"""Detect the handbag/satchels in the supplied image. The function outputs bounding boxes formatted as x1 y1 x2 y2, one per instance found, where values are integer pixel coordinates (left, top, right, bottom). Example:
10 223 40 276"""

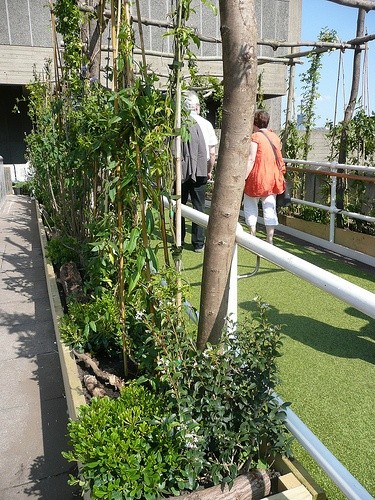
276 185 291 206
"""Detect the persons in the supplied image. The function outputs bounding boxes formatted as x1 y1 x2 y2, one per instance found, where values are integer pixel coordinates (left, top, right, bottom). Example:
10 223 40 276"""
170 89 217 253
244 109 286 246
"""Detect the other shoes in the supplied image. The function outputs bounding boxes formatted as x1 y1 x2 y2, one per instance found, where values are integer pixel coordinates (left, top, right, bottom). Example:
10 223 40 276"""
194 244 204 252
181 241 184 245
